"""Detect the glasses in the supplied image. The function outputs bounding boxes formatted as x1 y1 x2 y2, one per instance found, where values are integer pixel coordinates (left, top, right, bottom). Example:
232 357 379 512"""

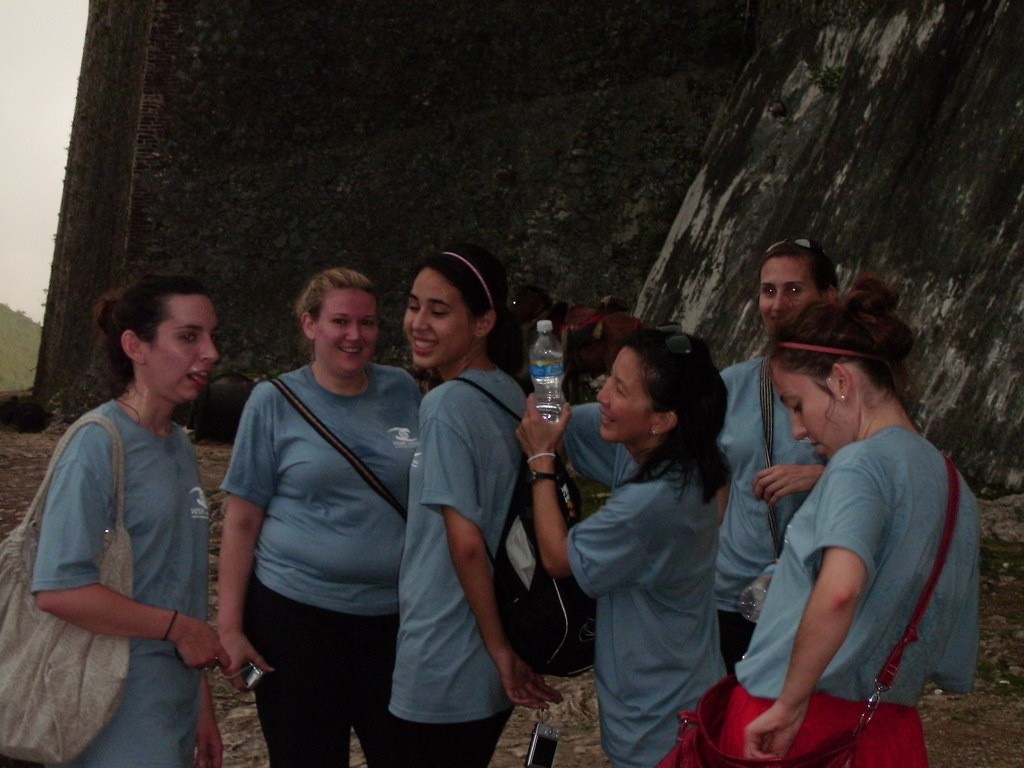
762 237 824 264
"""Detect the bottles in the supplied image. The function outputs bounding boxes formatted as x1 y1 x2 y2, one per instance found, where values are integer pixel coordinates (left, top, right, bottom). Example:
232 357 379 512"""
529 321 566 422
738 559 782 621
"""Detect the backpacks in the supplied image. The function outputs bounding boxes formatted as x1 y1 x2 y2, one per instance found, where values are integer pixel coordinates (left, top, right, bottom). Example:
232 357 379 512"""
450 377 598 677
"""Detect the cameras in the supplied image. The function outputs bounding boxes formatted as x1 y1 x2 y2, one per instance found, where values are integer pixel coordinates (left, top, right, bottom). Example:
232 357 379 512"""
524 723 560 768
242 662 264 690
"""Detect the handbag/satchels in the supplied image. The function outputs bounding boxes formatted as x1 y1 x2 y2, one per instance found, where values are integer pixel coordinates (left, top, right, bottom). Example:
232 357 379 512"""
655 672 856 768
0 415 135 764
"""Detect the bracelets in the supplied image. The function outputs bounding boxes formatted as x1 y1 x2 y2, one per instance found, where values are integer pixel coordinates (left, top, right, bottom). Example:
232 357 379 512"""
526 452 555 464
161 610 179 641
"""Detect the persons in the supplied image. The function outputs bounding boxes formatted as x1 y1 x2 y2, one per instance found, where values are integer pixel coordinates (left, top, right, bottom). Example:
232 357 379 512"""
378 247 564 768
512 319 740 768
685 233 840 673
13 269 235 768
213 267 427 768
723 297 986 768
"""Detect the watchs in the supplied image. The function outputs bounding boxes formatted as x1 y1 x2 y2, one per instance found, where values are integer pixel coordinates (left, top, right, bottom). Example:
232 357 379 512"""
525 469 556 486
